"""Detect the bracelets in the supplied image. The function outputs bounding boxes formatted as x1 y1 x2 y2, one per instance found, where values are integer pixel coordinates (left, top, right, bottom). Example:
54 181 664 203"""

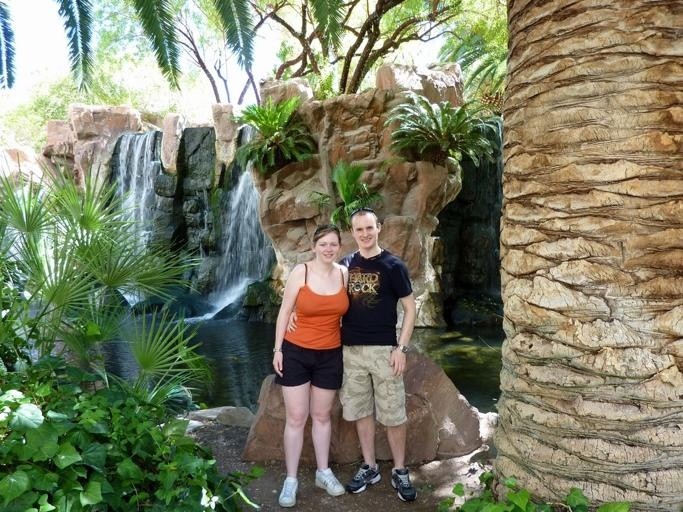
273 348 282 352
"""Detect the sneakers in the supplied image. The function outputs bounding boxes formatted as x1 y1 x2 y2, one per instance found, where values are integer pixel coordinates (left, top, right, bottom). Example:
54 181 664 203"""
391 468 418 502
314 468 346 496
347 464 382 494
278 476 299 508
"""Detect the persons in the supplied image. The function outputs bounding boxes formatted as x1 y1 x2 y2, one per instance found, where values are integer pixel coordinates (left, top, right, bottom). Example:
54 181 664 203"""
285 206 418 503
271 224 350 508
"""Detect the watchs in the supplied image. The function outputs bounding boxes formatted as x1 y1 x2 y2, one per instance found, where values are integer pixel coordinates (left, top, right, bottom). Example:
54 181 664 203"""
396 344 409 353
